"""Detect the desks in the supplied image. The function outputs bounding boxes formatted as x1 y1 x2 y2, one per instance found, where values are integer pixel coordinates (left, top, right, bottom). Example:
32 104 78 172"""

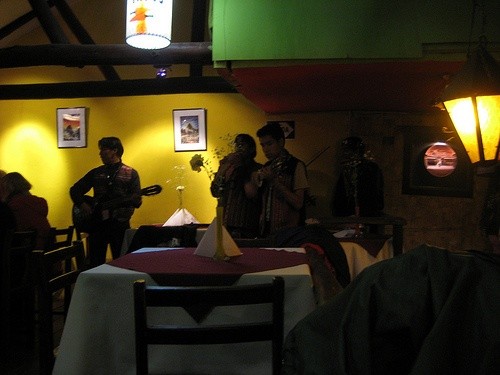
53 235 403 375
314 216 407 258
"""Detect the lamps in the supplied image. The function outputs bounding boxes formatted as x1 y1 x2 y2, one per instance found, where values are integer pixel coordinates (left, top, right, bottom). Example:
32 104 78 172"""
441 10 500 174
150 51 173 79
125 0 173 50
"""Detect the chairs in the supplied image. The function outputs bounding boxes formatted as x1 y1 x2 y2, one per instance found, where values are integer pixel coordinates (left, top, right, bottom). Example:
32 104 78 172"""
0 225 88 375
134 276 285 375
125 225 198 254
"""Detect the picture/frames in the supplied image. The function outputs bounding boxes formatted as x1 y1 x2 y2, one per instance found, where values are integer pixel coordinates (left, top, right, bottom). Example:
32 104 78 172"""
56 106 87 148
401 126 478 199
172 108 207 152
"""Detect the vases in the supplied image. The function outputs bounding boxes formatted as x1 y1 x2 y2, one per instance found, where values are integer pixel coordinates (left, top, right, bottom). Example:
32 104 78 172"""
213 207 230 262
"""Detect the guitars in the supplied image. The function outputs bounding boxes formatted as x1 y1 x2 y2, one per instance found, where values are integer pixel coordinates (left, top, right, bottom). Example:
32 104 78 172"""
72 185 162 232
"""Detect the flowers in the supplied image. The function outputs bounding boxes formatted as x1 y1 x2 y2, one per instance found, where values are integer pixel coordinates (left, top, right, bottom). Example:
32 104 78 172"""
189 133 248 206
165 166 186 209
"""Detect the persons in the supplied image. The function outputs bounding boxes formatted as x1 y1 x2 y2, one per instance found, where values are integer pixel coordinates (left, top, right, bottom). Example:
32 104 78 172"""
70 137 142 271
283 164 500 375
0 173 64 276
251 122 309 236
212 134 266 239
324 134 384 233
